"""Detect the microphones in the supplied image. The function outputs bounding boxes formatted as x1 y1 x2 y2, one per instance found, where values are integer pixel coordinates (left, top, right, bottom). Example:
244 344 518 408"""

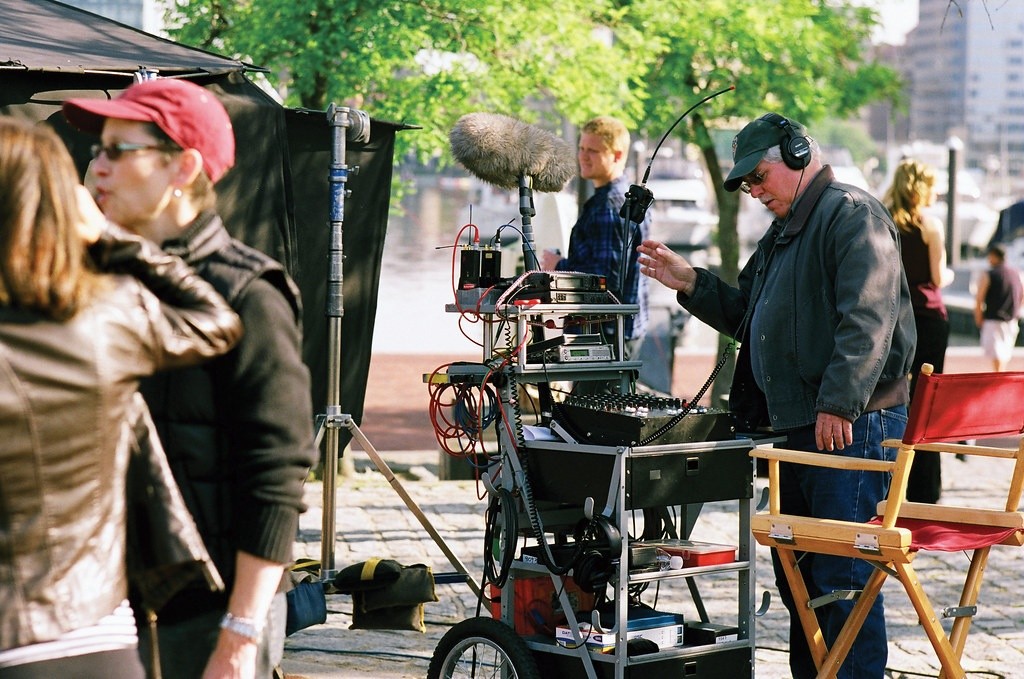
451 114 578 193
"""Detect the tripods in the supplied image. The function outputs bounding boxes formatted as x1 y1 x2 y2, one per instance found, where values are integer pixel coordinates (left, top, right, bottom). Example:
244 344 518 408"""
313 125 492 612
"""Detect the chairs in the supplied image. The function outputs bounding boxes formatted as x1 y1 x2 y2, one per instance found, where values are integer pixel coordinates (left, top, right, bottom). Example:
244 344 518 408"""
749 363 1023 679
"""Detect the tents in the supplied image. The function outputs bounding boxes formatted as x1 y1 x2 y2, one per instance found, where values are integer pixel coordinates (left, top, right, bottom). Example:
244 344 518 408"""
0 0 423 471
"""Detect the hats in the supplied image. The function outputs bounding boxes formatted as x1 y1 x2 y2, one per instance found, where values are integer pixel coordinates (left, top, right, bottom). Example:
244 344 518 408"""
63 79 238 186
723 114 802 192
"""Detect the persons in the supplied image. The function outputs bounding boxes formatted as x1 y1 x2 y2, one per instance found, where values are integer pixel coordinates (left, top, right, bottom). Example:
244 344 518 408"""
973 245 1024 377
635 112 915 679
539 116 652 403
877 160 954 501
0 74 318 679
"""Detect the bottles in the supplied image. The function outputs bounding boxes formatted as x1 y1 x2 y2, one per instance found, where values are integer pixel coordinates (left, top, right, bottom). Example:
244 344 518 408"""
632 547 683 573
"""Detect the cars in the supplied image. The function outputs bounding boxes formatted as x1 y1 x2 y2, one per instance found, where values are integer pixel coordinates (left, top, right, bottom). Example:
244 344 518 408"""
938 255 1023 347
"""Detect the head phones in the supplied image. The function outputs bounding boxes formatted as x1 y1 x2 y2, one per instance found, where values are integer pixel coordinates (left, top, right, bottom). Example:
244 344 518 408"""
760 112 812 170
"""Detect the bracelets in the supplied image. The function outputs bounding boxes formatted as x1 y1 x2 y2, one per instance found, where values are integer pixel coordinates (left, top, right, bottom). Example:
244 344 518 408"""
219 611 265 642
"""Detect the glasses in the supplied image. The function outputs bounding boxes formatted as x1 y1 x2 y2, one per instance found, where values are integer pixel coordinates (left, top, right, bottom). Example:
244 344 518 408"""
90 143 185 160
740 154 780 194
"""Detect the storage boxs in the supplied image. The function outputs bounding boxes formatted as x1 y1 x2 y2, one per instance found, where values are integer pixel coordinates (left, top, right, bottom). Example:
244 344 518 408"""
631 538 738 567
556 627 616 654
